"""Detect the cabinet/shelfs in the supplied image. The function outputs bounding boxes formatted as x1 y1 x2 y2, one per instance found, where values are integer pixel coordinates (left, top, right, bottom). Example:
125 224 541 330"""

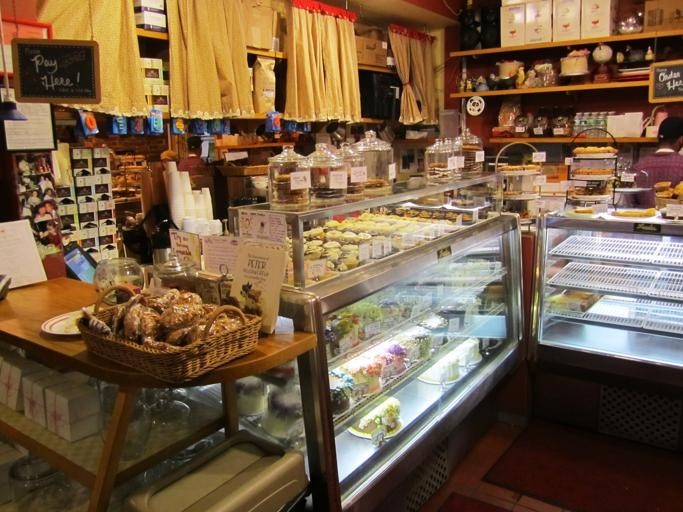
0 1 682 512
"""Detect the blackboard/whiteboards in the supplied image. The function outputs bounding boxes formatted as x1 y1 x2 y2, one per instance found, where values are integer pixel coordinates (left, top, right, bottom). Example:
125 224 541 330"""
11 38 101 104
648 59 683 104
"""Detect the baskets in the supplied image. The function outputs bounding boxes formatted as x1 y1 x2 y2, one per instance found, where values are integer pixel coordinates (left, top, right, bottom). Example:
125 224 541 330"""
75 282 264 387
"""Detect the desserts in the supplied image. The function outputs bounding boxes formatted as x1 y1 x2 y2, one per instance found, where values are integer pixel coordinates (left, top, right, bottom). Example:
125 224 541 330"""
288 204 491 285
288 259 491 447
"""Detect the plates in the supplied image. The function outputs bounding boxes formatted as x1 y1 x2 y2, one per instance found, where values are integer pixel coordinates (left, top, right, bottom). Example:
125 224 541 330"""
42 311 82 336
609 188 655 209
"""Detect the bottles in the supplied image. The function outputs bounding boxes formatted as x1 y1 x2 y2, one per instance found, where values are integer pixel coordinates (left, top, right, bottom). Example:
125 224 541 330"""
268 127 484 209
531 104 616 139
94 254 196 304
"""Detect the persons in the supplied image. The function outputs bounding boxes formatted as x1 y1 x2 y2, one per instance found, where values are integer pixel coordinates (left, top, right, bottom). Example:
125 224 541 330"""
121 202 170 266
248 124 275 164
176 136 226 194
18 157 62 252
623 115 682 209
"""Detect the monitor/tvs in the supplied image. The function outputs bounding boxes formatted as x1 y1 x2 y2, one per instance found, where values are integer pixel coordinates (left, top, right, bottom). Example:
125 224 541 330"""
63 243 97 284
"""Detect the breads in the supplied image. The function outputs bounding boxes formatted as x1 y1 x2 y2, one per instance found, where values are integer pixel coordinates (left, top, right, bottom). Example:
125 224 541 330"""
112 287 252 345
